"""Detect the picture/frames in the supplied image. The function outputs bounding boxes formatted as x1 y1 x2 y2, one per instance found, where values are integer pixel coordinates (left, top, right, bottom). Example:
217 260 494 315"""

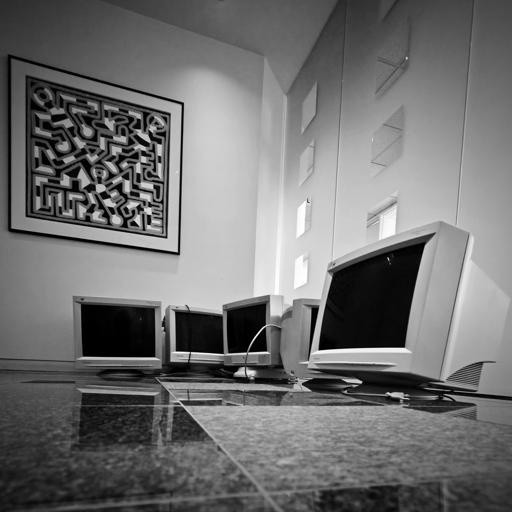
5 53 186 255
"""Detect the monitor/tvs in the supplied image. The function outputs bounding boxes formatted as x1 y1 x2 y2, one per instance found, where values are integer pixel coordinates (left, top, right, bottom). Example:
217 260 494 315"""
71 295 163 380
162 304 228 377
307 220 512 400
278 297 353 391
222 294 300 385
